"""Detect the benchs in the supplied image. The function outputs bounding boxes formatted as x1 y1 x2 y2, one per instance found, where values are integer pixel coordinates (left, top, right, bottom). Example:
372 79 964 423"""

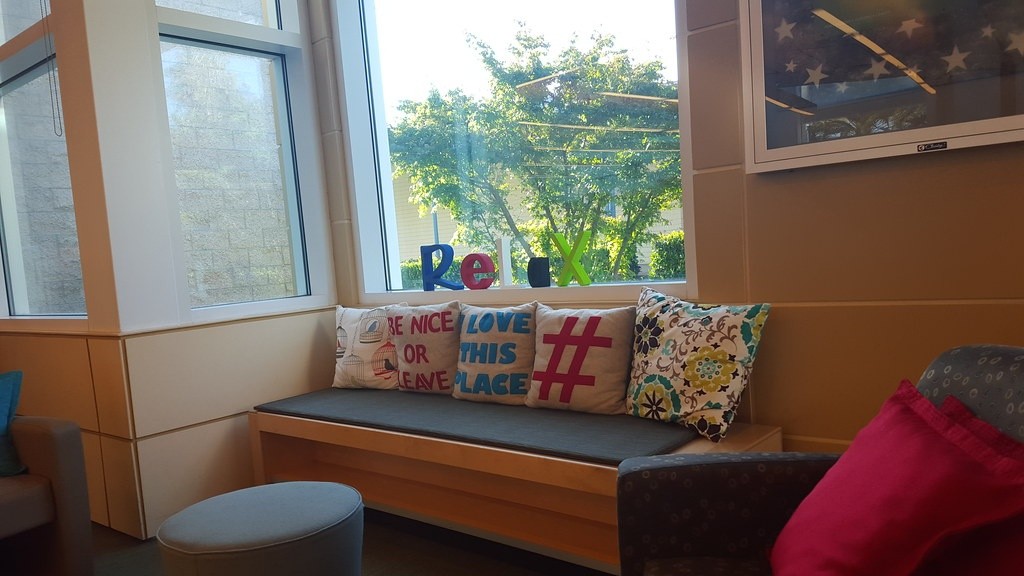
243 389 785 576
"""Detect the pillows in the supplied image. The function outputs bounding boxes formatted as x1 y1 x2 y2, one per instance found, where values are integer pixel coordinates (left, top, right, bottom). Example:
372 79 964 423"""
525 299 635 415
912 394 1024 576
0 367 25 478
763 375 1024 576
450 301 538 407
623 283 774 445
331 302 402 392
384 300 462 392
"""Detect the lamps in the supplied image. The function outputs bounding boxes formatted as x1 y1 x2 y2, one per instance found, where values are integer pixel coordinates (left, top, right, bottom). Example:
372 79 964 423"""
765 83 818 117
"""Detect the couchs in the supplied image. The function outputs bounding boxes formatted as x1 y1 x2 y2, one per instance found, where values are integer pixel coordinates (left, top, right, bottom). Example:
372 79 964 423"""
616 345 1024 576
0 414 96 576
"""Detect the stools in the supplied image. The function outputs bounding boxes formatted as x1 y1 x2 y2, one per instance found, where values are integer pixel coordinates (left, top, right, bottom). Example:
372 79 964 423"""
154 481 364 576
